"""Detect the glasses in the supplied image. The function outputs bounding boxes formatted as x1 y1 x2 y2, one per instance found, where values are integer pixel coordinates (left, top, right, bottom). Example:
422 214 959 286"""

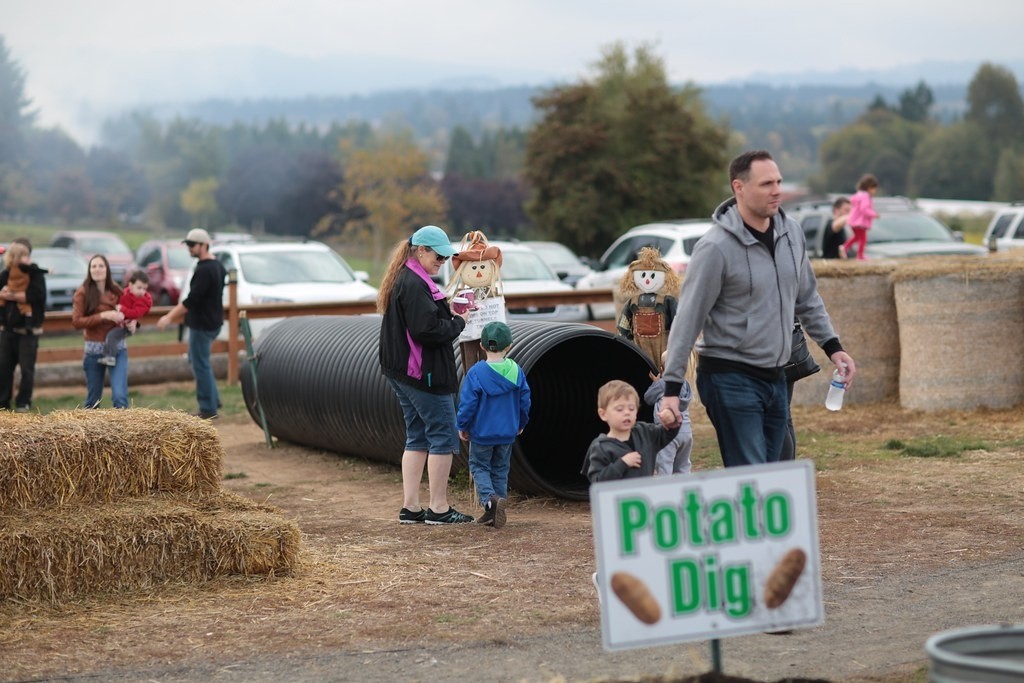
428 247 450 261
187 242 198 246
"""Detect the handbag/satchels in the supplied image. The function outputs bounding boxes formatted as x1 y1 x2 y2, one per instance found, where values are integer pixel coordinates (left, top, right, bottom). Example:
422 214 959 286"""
782 327 820 382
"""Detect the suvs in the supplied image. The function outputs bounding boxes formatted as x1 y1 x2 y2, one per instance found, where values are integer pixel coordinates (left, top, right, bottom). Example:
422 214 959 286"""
784 198 987 259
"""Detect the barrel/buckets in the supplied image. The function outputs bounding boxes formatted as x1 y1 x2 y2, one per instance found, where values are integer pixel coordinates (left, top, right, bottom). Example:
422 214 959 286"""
926 624 1024 683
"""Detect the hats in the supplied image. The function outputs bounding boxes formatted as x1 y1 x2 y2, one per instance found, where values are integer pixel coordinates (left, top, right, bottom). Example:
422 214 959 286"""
180 227 211 246
411 225 460 257
481 322 513 351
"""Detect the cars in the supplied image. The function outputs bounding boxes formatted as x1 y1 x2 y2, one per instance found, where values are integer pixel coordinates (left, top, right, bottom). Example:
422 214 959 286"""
177 241 378 339
522 240 594 288
50 228 136 288
429 240 584 325
983 200 1024 255
27 247 91 313
575 215 715 321
123 233 254 307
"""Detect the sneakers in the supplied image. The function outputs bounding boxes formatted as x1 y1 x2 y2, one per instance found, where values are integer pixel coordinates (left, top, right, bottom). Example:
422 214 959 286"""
398 507 427 523
424 504 474 525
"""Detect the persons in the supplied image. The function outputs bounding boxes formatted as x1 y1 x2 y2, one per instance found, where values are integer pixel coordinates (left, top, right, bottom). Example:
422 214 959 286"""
378 223 474 524
0 239 48 412
455 320 531 528
581 380 684 604
838 174 880 259
659 150 855 636
72 254 153 408
821 197 851 259
444 229 504 314
643 350 693 476
156 227 227 421
616 247 699 381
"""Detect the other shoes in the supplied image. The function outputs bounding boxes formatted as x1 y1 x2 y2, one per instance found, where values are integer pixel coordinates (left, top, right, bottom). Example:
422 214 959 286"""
190 409 218 421
97 356 116 366
838 245 847 259
215 399 223 409
490 495 507 528
477 508 494 523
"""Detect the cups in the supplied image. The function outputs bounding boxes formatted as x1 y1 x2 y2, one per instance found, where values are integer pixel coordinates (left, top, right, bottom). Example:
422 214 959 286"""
453 297 468 315
457 289 476 311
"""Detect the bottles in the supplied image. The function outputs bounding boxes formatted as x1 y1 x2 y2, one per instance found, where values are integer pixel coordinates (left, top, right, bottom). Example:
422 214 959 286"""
825 361 848 411
